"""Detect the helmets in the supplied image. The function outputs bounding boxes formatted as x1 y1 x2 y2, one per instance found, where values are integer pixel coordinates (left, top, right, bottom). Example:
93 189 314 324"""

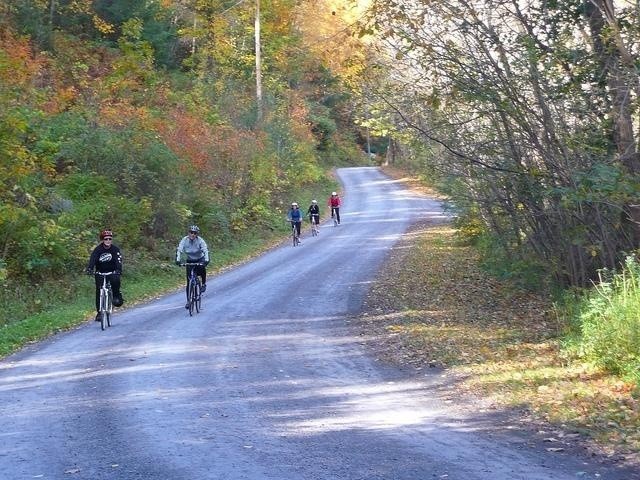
189 225 200 234
100 230 113 241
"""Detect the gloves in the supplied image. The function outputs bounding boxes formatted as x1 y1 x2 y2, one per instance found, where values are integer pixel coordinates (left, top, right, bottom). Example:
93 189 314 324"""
87 267 94 275
112 269 121 276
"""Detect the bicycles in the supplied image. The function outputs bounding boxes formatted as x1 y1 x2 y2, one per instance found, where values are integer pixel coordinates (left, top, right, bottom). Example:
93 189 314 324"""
85 270 121 330
179 262 204 316
307 214 320 237
329 206 340 228
288 219 300 247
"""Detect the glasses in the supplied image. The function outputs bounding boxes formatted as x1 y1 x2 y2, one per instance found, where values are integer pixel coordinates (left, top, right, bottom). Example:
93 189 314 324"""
104 238 112 240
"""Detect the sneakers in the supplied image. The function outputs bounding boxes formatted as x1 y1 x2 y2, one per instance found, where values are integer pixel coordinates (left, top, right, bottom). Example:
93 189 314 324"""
112 293 123 307
200 284 206 292
95 311 104 321
185 301 190 309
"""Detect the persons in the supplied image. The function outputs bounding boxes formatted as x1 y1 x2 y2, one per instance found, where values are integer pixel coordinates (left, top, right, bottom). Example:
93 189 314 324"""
327 191 341 224
90 231 123 321
288 203 302 238
306 200 320 233
175 225 210 308
